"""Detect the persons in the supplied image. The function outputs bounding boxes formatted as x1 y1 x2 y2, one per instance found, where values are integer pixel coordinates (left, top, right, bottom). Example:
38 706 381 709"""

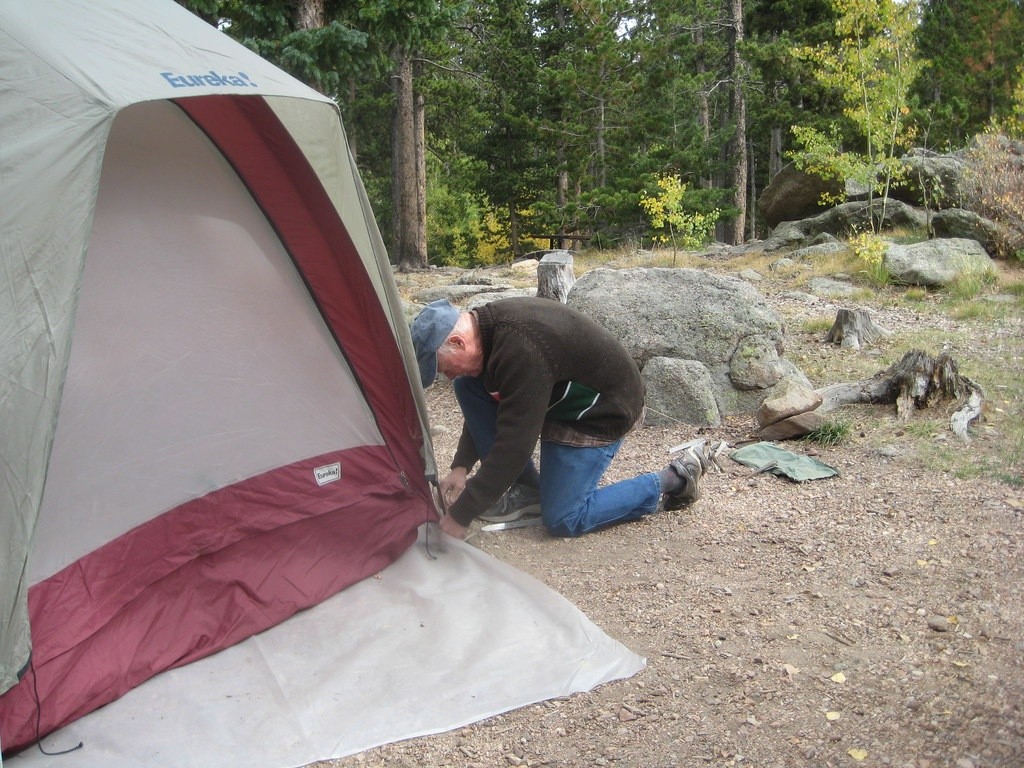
410 296 708 539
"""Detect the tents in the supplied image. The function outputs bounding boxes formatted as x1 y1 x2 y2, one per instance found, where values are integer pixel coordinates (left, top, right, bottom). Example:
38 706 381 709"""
0 0 444 761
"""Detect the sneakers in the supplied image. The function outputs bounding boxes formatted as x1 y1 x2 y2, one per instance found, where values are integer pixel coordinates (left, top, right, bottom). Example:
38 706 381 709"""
662 447 708 511
478 483 541 522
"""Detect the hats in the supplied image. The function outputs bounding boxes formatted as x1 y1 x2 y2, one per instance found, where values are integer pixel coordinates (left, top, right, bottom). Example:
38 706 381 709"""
410 298 460 389
